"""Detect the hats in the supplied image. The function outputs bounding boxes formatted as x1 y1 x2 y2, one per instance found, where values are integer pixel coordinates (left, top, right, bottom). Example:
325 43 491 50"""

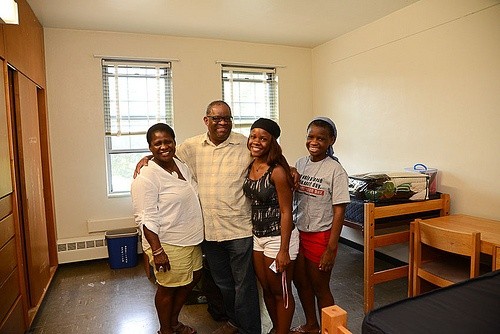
251 118 281 139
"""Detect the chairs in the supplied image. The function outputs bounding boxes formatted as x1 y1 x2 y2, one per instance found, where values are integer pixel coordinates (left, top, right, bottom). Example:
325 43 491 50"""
412 219 481 298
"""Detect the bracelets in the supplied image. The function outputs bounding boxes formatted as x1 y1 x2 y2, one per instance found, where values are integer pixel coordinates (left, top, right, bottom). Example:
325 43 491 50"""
153 247 164 256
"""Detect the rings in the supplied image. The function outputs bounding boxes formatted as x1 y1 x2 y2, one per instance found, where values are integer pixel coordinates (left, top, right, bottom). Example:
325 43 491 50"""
160 266 163 269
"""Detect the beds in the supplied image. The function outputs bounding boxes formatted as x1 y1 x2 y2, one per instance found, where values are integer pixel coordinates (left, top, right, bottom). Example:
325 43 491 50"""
322 269 500 334
342 190 451 311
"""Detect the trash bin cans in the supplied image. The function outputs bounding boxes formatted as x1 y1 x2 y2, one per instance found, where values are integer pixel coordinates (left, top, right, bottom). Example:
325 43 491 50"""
105 227 140 269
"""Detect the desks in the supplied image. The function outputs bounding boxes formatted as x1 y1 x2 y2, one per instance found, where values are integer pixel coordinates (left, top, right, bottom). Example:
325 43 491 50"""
408 215 500 299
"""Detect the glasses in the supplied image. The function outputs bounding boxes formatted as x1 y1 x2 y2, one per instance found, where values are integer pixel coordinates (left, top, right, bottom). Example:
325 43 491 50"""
207 115 233 122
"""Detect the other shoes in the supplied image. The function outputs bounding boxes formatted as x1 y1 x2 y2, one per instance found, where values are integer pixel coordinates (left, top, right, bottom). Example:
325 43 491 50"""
290 324 320 334
170 321 197 334
212 321 238 334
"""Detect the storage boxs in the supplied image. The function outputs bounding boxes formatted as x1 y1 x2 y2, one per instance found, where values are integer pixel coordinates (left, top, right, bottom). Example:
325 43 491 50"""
405 168 439 193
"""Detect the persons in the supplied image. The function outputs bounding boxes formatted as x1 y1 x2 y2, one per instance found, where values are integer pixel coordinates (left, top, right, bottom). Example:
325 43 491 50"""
132 100 300 334
242 118 300 334
292 117 351 334
129 123 205 334
